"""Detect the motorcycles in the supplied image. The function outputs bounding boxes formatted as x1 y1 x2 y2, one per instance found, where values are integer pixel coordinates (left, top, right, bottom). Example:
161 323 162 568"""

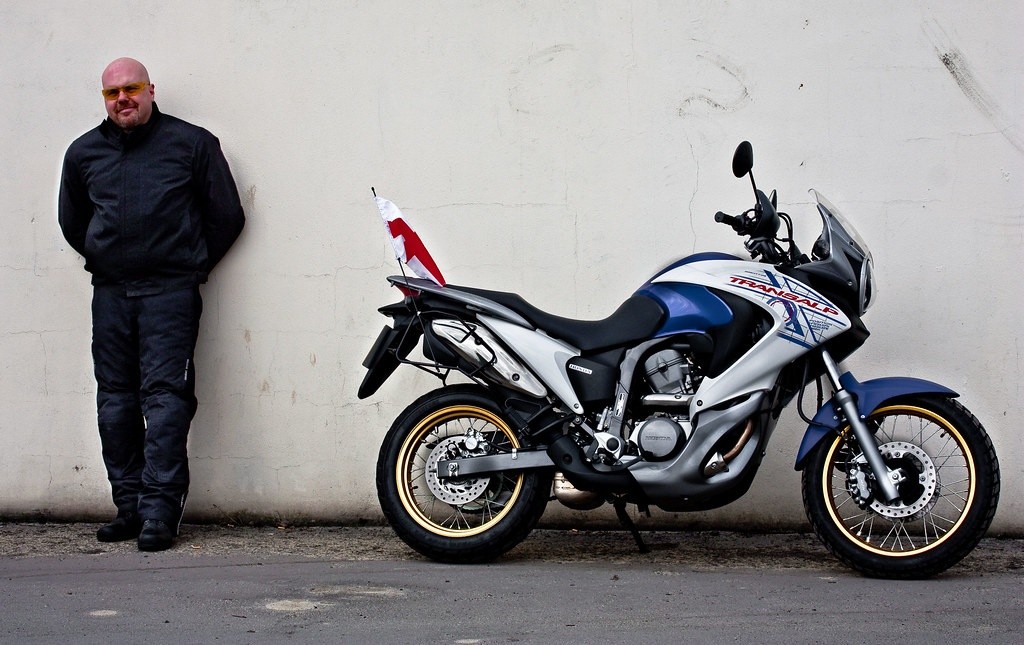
357 141 1002 579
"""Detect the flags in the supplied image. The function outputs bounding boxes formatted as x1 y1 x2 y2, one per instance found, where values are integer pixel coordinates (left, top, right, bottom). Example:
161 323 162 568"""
377 197 446 286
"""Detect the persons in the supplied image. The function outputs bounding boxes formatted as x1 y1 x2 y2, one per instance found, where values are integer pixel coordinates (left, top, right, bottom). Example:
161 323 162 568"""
58 57 246 551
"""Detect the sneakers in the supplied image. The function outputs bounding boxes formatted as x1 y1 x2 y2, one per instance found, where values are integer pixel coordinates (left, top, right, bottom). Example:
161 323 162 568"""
96 511 141 542
138 518 176 551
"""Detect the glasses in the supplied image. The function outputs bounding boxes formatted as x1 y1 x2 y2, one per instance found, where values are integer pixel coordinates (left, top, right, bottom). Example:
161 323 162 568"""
102 81 149 100
